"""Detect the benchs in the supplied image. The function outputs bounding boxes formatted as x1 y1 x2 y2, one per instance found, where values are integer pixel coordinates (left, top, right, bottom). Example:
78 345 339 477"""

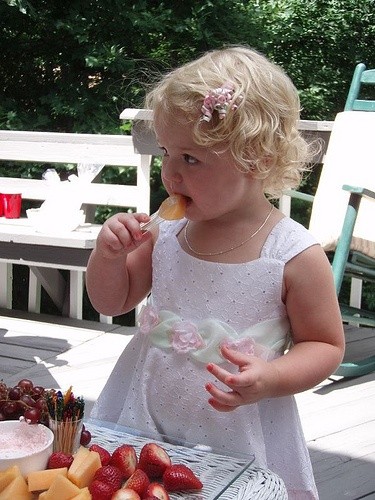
0 128 152 325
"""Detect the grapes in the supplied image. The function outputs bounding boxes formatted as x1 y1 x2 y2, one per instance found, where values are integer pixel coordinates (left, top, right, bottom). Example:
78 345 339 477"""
0 379 92 445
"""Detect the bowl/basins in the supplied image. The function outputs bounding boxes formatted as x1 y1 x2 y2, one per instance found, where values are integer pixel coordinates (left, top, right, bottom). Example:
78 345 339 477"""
27 208 84 232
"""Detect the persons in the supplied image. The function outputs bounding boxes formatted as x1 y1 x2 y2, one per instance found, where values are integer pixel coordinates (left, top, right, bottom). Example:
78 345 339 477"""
82 44 348 500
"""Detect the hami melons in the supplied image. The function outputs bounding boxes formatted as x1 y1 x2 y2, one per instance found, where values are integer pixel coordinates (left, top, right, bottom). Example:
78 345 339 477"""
157 194 186 220
0 445 102 500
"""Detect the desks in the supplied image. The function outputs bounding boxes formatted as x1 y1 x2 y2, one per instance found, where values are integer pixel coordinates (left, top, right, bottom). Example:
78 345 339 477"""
0 413 289 500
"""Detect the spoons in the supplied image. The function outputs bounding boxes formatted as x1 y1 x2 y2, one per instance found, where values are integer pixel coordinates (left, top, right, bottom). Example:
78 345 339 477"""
131 195 187 242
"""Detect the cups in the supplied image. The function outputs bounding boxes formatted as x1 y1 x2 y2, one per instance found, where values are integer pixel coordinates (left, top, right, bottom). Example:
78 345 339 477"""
49 416 83 456
0 420 55 484
4 194 22 218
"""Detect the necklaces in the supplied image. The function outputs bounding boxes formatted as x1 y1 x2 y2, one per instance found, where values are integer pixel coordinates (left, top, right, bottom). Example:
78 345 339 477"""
183 203 275 257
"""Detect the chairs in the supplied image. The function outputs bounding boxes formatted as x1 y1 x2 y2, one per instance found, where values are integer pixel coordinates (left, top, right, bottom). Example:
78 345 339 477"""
278 62 375 378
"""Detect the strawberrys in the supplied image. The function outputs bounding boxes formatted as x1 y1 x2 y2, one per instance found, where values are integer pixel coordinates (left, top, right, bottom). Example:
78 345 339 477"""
48 443 204 500
